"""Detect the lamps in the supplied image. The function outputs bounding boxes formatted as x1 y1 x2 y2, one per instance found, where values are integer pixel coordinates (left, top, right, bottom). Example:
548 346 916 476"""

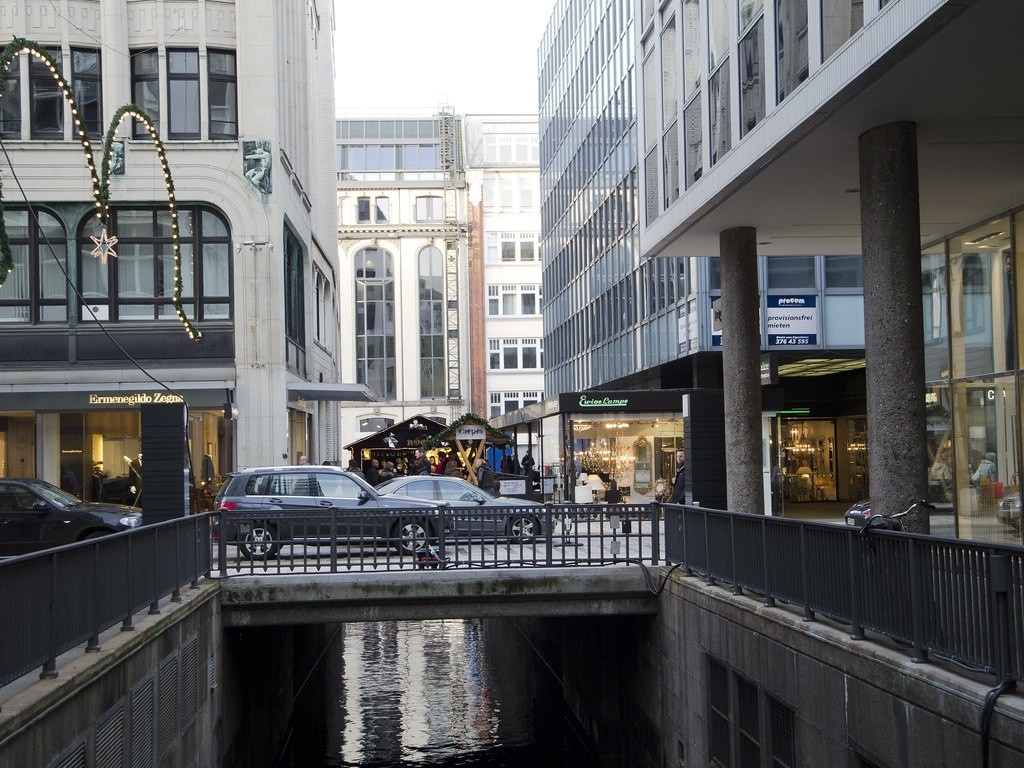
782 421 837 501
572 420 683 505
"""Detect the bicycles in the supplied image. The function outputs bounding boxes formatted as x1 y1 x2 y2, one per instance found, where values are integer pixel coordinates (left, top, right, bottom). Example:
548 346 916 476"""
856 501 938 535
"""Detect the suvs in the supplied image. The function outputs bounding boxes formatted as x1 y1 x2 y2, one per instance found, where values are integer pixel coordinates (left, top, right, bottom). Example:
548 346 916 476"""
212 466 453 560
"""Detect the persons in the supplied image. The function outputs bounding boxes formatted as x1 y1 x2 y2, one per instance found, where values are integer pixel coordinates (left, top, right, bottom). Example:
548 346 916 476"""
366 446 535 494
348 458 366 482
670 447 687 505
931 451 951 480
977 451 998 483
298 455 310 466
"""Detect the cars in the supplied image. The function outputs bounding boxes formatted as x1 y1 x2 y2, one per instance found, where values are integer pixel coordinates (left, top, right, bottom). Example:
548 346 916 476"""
369 476 557 544
0 477 145 559
